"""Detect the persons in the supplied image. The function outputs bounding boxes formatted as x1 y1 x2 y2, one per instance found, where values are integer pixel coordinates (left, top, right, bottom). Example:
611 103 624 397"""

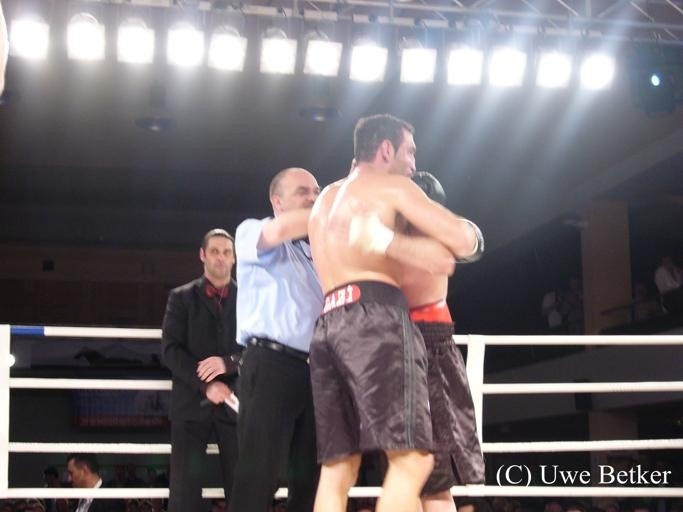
225 168 324 512
539 255 683 328
0 455 682 512
159 229 245 512
347 170 486 512
308 114 485 512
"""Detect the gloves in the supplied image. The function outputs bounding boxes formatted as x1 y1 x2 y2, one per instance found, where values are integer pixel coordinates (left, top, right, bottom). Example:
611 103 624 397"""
411 170 447 204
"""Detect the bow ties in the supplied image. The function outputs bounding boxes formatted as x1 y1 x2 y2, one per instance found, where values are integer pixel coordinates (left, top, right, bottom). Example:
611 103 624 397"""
205 284 231 299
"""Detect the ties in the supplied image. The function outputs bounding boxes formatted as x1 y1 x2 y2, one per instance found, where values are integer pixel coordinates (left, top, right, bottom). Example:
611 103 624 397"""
78 498 88 511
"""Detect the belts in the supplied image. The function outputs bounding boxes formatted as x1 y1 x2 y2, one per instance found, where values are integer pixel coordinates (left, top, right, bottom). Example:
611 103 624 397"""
244 337 311 366
